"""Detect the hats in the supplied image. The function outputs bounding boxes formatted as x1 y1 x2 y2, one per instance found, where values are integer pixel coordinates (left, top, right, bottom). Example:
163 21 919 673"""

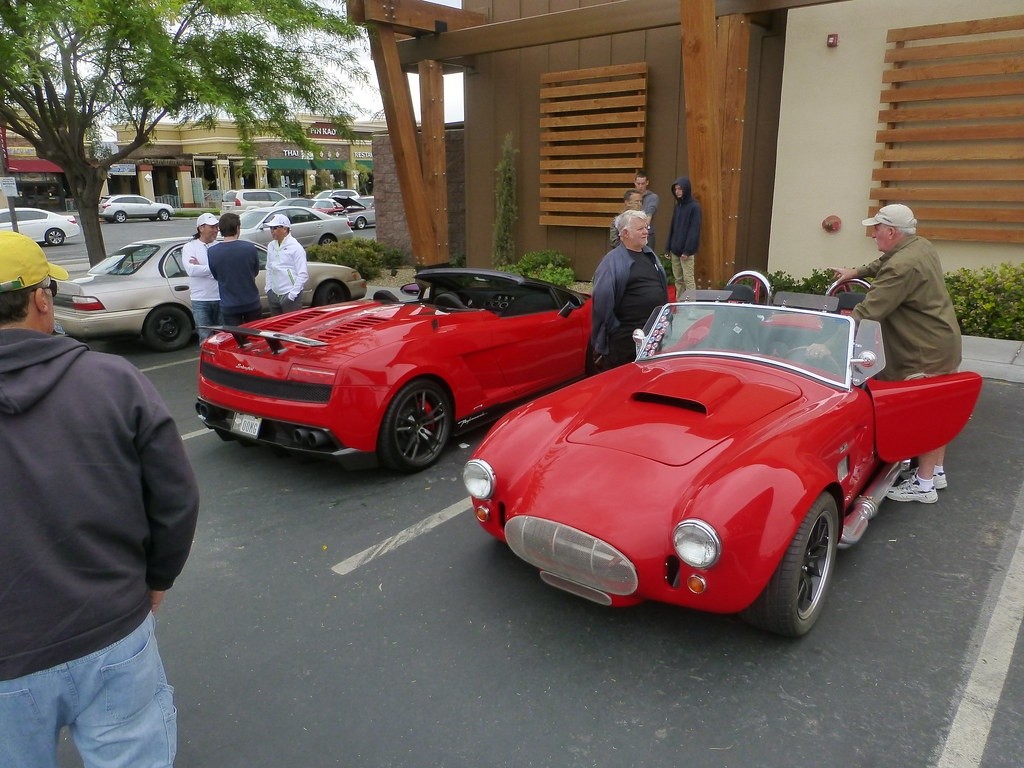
1 231 69 292
862 204 918 228
264 214 291 230
197 213 220 228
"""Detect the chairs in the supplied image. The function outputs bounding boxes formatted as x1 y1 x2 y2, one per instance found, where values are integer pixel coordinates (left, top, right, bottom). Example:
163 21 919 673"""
373 291 400 303
433 294 465 309
821 290 873 361
699 283 759 351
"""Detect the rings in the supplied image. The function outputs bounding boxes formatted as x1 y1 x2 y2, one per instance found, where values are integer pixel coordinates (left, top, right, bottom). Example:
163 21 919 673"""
815 353 818 355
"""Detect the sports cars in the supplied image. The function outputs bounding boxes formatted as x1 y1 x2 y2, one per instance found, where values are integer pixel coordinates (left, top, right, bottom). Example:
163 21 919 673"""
194 260 680 473
462 270 986 638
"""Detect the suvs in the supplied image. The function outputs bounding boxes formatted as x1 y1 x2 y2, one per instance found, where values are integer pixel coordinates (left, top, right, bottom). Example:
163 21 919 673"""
98 194 175 223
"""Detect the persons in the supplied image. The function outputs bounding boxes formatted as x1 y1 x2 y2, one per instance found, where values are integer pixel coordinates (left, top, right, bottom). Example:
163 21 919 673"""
263 214 310 316
207 213 262 326
590 209 669 366
182 213 226 344
634 172 658 250
0 230 201 768
664 176 702 320
610 189 643 248
806 203 963 503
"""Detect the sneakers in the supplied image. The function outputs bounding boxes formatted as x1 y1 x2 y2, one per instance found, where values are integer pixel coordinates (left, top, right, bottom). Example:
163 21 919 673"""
886 476 938 503
900 468 948 489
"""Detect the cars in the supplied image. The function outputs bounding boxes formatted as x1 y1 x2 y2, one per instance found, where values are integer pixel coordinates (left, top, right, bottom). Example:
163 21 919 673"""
0 207 81 246
40 235 367 350
272 189 377 230
214 206 354 251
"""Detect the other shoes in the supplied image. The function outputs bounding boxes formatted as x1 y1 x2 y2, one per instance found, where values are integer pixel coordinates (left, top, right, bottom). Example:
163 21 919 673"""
688 310 697 320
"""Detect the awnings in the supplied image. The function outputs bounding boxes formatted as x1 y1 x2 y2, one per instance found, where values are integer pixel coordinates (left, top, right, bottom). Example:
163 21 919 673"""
312 160 350 169
268 159 312 170
7 160 64 172
356 160 373 169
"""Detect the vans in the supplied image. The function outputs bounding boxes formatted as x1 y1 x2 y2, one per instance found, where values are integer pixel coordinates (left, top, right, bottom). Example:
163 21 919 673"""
219 188 290 215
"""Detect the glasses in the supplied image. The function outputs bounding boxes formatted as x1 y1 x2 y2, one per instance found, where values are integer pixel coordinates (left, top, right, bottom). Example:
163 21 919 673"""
41 280 57 297
